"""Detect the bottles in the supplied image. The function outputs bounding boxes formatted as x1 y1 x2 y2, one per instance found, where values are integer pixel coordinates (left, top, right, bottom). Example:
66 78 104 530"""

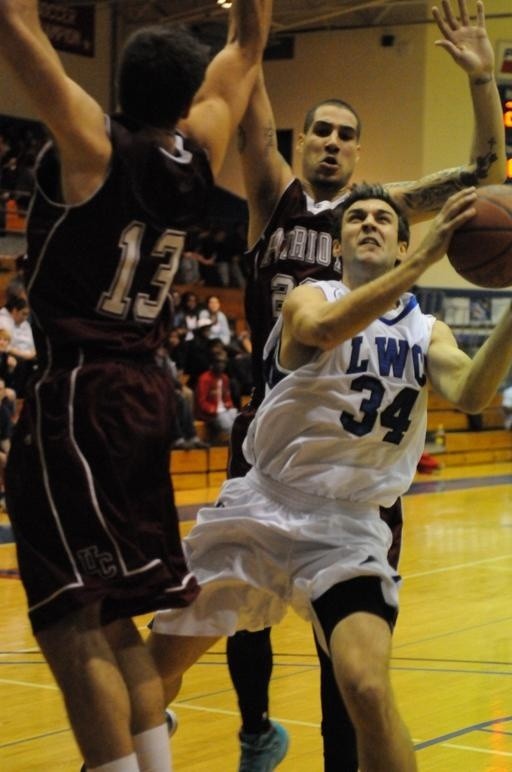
434 423 446 446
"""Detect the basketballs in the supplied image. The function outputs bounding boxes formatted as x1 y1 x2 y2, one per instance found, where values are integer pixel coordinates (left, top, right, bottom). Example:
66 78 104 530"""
446 181 512 287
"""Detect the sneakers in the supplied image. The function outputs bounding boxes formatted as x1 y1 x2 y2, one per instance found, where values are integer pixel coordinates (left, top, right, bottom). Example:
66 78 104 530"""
237 720 291 772
80 708 177 772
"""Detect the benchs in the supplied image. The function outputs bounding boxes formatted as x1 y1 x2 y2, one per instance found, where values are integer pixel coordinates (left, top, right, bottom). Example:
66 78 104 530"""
171 297 253 490
423 378 511 483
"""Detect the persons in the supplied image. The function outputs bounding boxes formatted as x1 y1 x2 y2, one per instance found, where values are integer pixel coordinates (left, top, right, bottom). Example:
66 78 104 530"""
224 0 512 769
3 1 275 770
158 290 254 451
81 179 509 771
1 288 38 468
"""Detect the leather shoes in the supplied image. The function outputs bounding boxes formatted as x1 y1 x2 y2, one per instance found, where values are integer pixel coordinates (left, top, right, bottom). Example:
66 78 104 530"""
191 439 210 448
173 441 191 450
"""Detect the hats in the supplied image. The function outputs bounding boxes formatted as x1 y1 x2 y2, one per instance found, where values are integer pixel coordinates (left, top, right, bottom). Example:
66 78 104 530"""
192 319 215 333
